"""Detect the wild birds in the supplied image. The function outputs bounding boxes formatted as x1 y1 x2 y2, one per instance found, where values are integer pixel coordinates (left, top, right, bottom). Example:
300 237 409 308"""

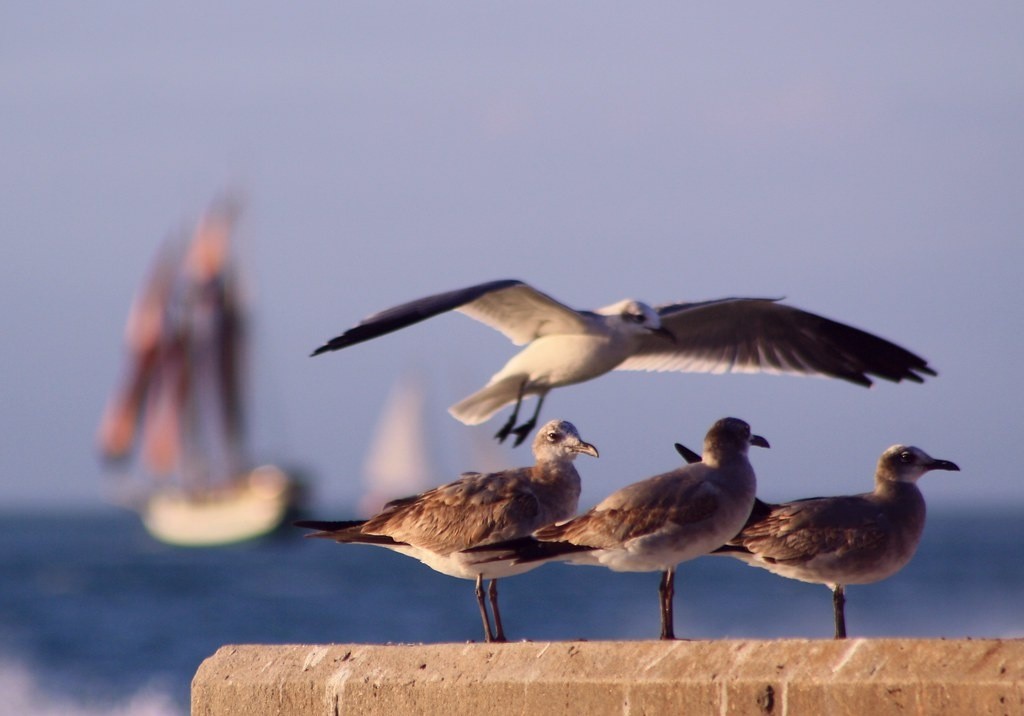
302 420 599 640
458 418 771 639
310 274 940 444
673 442 961 640
